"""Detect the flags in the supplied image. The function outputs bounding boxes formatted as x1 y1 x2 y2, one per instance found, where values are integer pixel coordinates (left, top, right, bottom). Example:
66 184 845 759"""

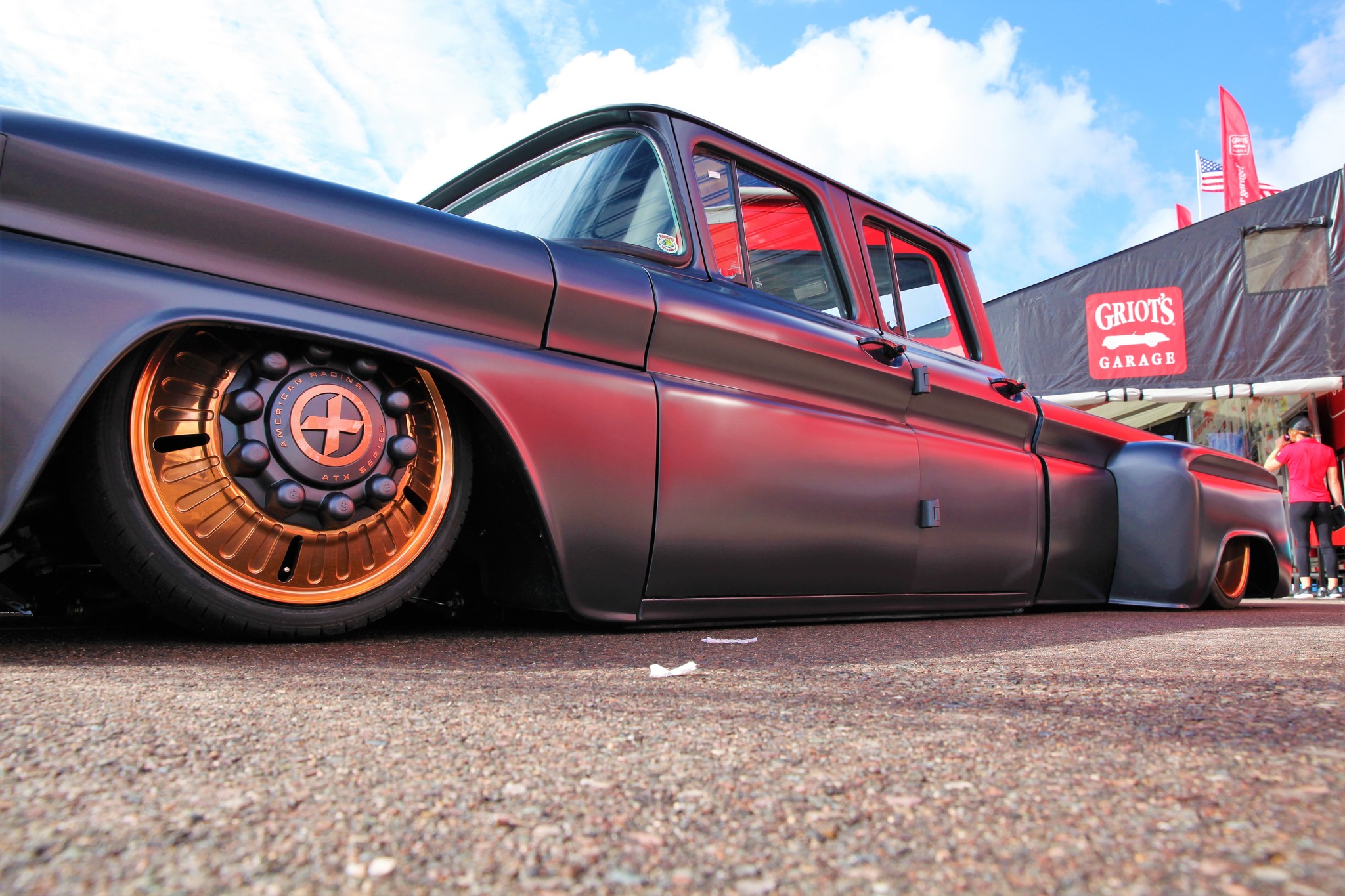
1175 203 1193 229
1197 154 1282 199
1218 84 1261 212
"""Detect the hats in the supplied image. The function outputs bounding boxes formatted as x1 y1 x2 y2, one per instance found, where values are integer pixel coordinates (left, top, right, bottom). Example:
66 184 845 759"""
1278 416 1313 433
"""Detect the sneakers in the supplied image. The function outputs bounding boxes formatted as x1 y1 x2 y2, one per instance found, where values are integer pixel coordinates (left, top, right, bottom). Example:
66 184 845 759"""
1293 587 1314 598
1326 586 1342 599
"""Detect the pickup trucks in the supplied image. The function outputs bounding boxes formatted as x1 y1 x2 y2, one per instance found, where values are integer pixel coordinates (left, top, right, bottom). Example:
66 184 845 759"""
1 101 1295 625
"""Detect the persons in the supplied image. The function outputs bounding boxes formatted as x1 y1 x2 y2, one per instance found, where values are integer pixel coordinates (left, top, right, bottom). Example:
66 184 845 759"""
1261 416 1345 598
